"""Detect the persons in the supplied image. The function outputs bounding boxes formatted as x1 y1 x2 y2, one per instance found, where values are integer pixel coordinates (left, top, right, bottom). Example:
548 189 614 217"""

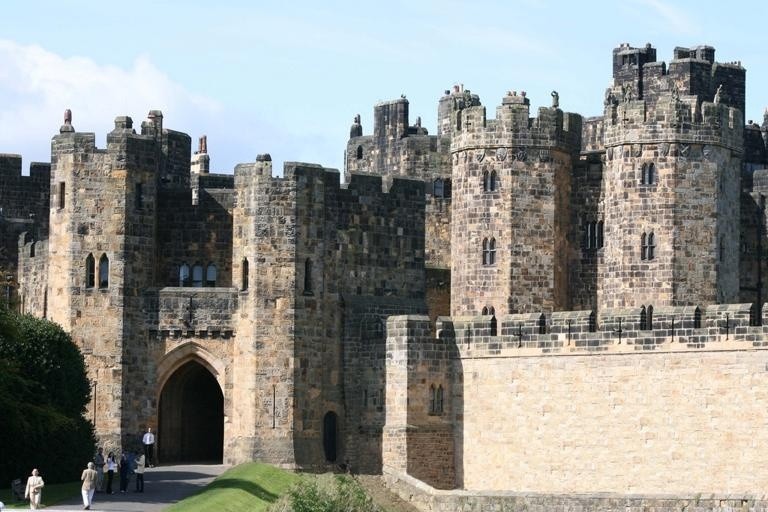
143 427 155 468
81 462 98 510
25 468 44 510
96 448 145 495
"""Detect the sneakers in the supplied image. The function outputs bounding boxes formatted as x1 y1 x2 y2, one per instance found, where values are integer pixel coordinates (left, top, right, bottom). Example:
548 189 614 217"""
96 489 116 494
84 505 91 510
120 489 144 495
